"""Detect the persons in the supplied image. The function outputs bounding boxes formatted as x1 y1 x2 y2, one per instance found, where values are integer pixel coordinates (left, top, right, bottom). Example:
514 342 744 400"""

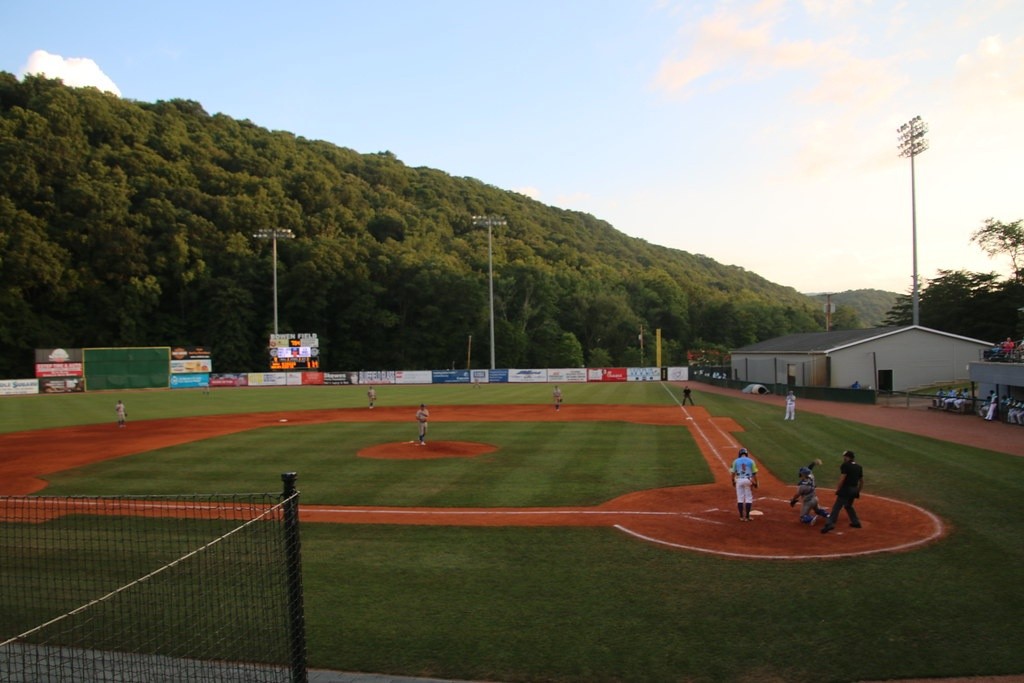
554 386 561 411
203 377 211 394
978 390 1024 425
987 337 1024 360
730 448 759 521
821 450 864 533
784 391 796 420
473 376 480 387
852 381 861 389
789 459 830 526
935 387 968 410
116 399 127 427
682 385 694 406
416 404 429 445
368 387 376 409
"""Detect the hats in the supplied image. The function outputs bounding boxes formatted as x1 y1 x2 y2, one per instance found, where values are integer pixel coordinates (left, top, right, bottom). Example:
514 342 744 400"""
421 404 426 407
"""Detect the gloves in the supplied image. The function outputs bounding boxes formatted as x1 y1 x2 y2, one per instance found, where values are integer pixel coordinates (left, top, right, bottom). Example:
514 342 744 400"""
790 498 799 507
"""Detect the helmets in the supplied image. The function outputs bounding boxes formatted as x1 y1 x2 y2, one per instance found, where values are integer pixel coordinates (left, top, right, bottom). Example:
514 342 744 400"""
799 467 811 477
738 448 748 457
843 450 855 459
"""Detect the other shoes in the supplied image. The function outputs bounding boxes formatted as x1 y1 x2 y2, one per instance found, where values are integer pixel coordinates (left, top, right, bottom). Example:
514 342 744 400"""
810 516 818 526
369 406 374 410
821 523 834 533
739 518 754 521
421 441 425 445
849 522 861 529
827 515 830 518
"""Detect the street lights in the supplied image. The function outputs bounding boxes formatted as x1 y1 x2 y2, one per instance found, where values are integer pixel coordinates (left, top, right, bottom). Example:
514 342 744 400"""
896 116 931 326
471 214 506 369
253 227 296 336
